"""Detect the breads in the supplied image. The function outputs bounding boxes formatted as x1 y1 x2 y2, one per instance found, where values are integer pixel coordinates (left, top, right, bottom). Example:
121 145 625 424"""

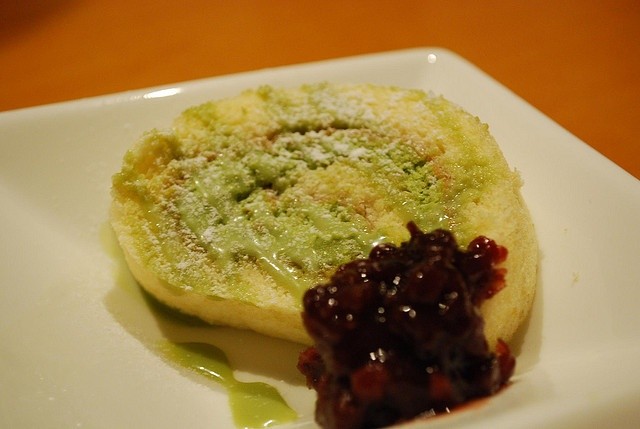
108 83 538 355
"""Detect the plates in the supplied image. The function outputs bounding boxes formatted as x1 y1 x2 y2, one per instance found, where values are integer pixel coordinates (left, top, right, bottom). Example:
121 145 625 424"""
0 46 640 428
